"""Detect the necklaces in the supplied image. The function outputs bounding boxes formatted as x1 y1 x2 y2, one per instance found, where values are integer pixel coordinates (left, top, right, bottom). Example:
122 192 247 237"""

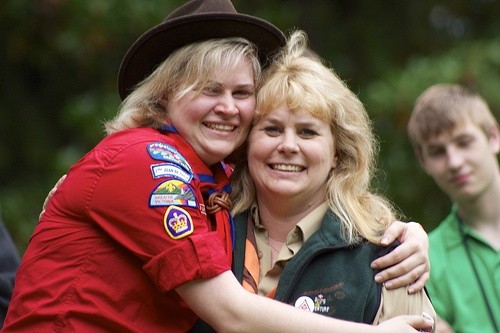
455 212 500 333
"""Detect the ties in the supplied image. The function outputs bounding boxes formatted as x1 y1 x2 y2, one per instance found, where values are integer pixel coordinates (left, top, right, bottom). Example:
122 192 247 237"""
204 188 233 269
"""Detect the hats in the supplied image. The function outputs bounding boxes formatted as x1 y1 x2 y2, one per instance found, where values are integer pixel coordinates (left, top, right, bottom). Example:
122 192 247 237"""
118 0 288 103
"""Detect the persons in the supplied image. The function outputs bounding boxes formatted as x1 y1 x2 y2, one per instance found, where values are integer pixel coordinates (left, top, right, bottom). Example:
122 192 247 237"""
39 31 436 333
408 83 500 333
0 0 433 333
